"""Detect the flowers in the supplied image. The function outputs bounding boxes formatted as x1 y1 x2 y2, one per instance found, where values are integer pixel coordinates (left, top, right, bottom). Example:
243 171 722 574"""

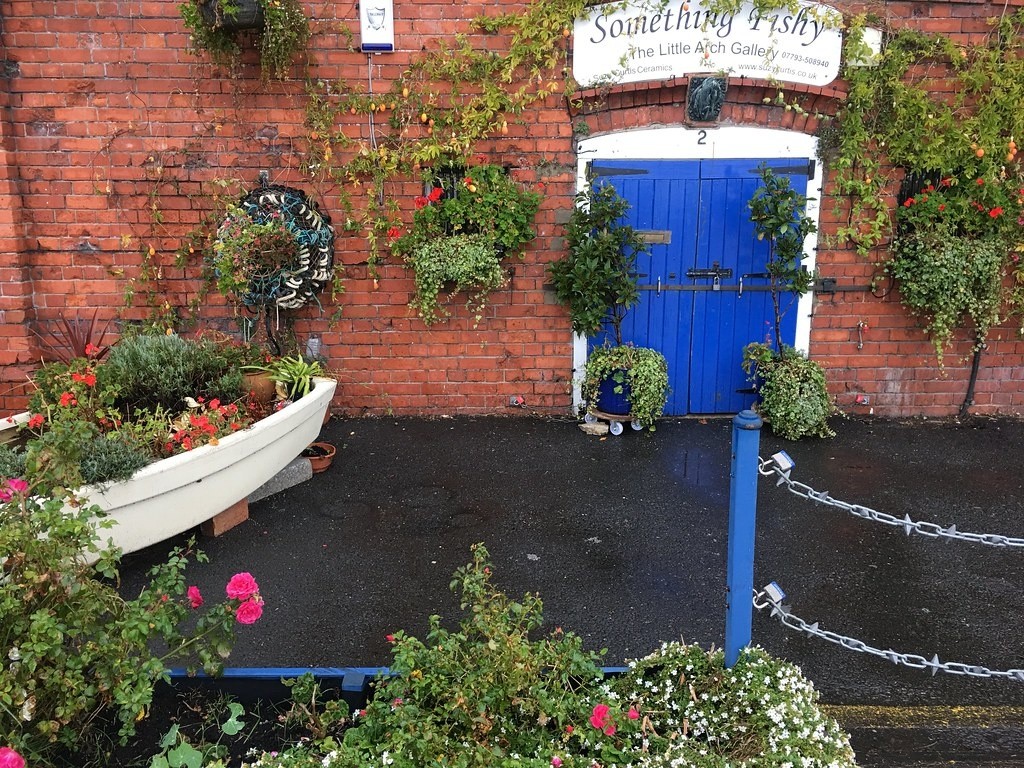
0 473 864 768
158 396 241 449
210 208 296 288
8 344 122 438
898 165 1018 297
386 154 545 329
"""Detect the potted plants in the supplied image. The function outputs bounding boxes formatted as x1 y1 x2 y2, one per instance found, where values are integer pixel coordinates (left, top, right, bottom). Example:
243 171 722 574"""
545 165 669 417
741 158 844 439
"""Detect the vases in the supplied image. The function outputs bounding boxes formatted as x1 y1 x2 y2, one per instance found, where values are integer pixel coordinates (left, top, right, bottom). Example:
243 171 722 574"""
242 371 277 403
307 444 336 474
